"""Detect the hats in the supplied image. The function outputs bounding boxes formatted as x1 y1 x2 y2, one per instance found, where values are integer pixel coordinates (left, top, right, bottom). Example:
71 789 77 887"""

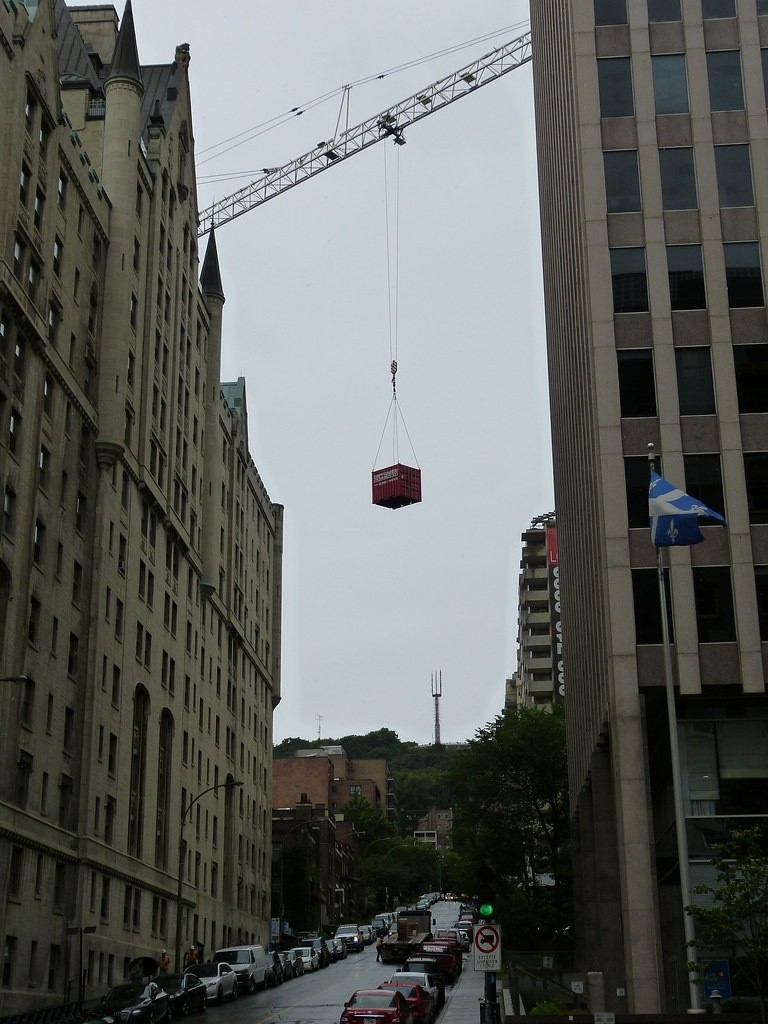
190 945 195 950
162 949 166 953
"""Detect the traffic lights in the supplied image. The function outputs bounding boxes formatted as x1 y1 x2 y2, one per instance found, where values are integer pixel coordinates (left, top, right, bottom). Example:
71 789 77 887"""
479 901 495 921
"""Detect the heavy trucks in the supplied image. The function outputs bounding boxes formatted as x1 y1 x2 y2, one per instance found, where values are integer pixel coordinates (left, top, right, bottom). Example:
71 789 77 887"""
379 909 437 965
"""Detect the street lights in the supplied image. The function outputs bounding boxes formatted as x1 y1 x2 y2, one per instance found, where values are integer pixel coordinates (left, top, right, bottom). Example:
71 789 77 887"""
175 780 246 973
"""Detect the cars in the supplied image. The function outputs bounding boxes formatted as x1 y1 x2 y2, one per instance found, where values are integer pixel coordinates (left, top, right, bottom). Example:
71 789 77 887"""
339 902 478 1024
90 889 457 1024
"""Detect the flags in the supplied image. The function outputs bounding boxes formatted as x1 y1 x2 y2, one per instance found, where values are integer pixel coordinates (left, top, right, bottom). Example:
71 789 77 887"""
649 472 728 546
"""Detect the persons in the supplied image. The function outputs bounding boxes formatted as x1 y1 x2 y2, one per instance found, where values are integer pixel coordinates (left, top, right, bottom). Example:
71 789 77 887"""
376 935 384 962
158 948 170 975
184 945 197 968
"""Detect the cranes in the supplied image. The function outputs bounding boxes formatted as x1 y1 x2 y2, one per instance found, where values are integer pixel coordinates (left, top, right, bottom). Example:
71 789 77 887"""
196 18 536 511
